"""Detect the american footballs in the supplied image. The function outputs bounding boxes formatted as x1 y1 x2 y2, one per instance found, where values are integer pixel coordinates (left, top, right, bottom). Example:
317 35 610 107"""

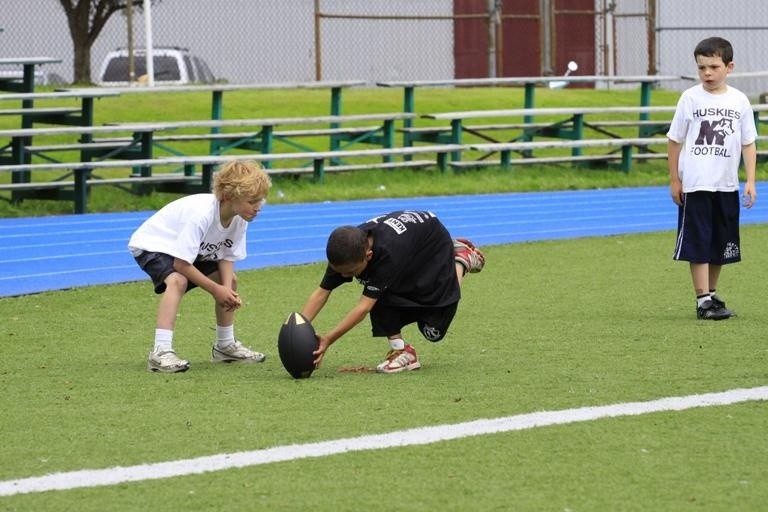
278 312 319 379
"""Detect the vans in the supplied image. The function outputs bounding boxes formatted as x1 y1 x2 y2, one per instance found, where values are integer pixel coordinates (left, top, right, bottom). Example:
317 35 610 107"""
98 48 215 87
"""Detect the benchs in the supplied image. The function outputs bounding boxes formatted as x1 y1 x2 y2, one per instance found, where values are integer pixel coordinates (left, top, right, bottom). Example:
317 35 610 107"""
1 71 768 212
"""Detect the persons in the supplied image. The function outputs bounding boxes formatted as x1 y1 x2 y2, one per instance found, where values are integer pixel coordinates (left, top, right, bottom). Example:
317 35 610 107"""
302 207 486 373
535 68 573 88
125 156 275 374
667 35 758 321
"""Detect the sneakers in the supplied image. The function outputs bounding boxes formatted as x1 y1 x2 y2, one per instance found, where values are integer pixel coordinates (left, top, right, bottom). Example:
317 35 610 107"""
711 295 737 318
376 337 421 374
148 349 190 373
696 299 730 321
452 238 485 273
211 340 265 364
